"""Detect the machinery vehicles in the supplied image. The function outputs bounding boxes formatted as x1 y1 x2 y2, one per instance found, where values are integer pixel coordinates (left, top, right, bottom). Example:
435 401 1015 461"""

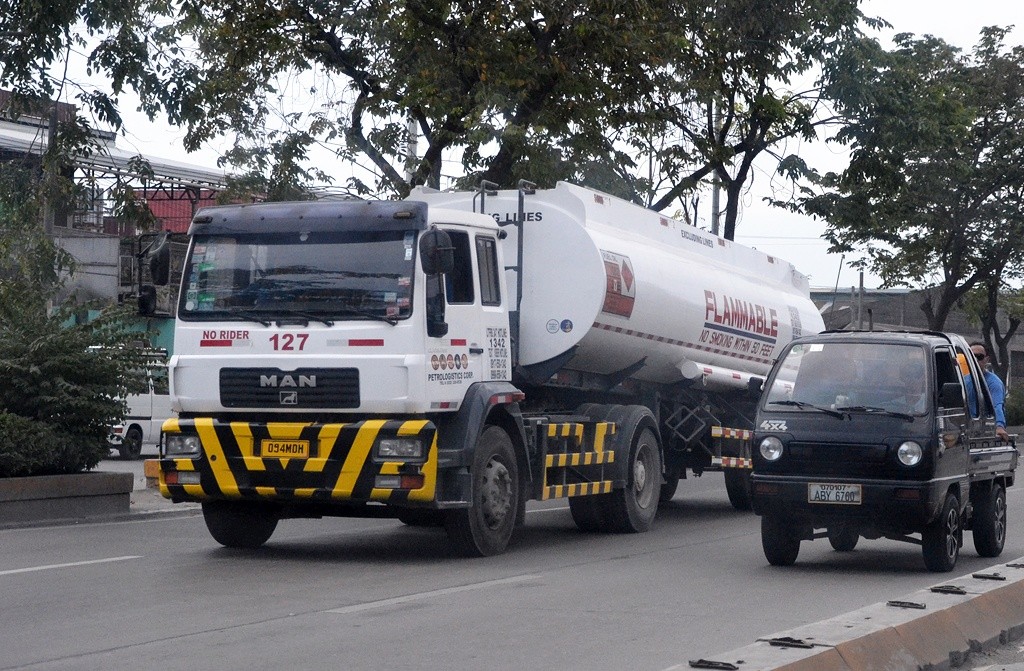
137 180 826 559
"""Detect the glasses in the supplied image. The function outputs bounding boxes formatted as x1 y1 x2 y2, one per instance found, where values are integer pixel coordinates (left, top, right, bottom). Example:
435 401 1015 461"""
975 353 987 360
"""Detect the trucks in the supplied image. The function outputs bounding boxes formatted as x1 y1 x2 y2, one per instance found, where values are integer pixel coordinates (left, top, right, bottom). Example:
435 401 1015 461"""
751 330 1019 572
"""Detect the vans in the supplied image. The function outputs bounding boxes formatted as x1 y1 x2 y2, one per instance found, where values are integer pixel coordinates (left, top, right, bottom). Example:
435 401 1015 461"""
86 346 172 460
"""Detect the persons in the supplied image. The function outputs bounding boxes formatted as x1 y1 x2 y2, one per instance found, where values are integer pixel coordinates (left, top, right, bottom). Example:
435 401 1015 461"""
964 342 1009 442
883 357 927 414
